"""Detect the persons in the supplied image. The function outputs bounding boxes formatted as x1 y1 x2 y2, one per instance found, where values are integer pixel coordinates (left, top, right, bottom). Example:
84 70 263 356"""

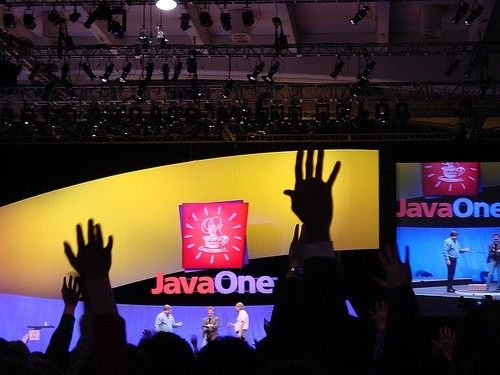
155 305 180 332
202 306 219 345
227 302 249 338
0 146 500 375
486 232 500 291
443 231 470 293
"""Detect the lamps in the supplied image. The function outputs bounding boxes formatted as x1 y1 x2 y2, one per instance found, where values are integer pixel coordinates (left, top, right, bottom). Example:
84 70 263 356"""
0 0 289 90
443 51 494 95
0 95 413 133
359 56 376 80
351 0 370 26
450 0 485 25
330 55 345 79
